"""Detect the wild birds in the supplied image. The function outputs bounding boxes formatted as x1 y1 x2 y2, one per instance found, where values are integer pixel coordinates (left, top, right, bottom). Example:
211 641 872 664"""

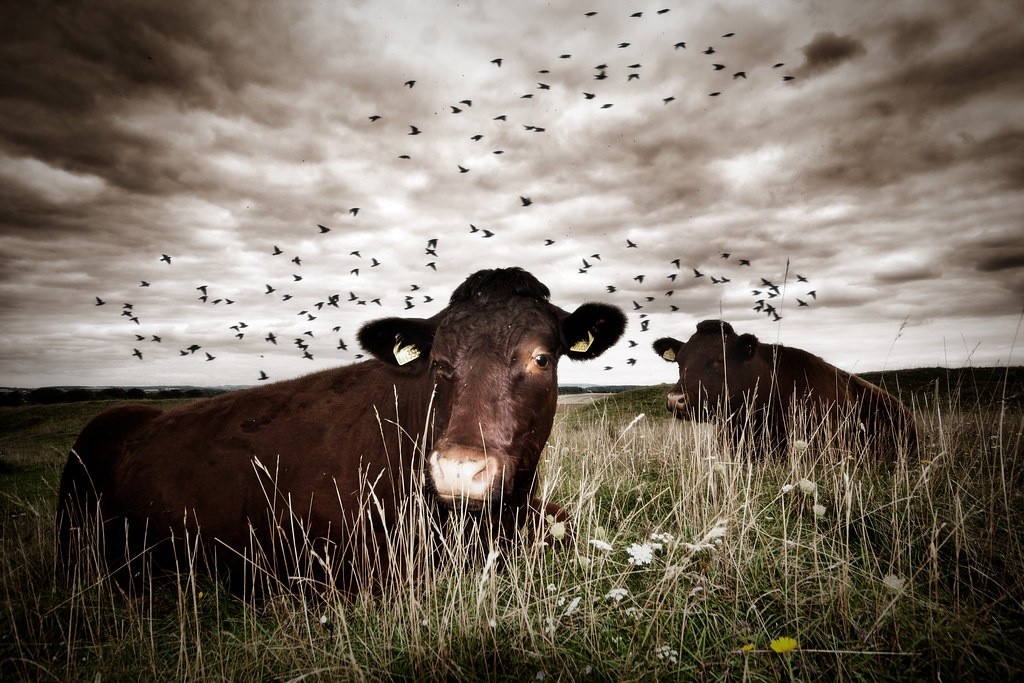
367 9 797 173
95 208 439 380
470 194 818 372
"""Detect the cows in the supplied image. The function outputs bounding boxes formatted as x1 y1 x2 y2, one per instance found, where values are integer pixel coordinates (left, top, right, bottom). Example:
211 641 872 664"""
57 268 626 605
654 319 916 471
